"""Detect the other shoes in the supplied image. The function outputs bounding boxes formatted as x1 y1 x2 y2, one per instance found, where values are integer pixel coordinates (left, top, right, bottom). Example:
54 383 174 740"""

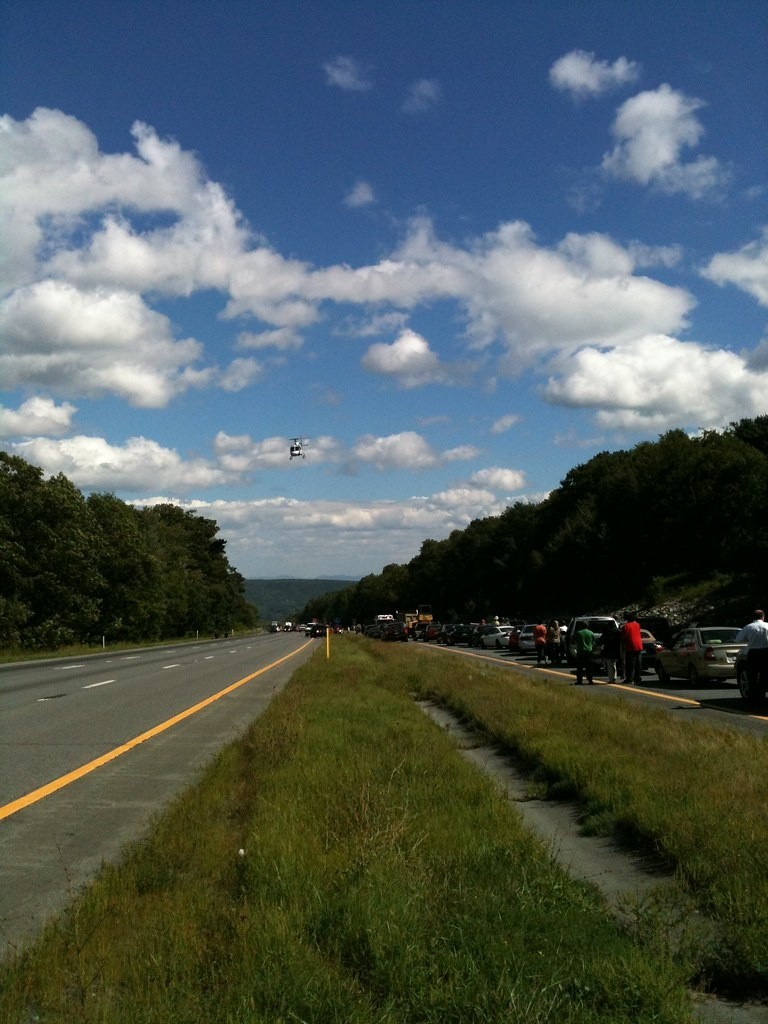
575 681 582 685
589 681 593 684
623 678 633 683
634 677 641 683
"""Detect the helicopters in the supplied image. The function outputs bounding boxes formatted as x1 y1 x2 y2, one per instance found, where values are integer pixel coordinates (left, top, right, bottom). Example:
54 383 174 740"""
288 435 308 461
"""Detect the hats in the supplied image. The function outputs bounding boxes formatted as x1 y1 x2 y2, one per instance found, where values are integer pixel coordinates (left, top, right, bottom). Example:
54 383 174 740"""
494 616 499 620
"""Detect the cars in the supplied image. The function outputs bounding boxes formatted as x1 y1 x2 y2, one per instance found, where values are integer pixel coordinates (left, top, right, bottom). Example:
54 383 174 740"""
653 626 744 690
269 620 328 638
732 643 768 700
361 604 664 671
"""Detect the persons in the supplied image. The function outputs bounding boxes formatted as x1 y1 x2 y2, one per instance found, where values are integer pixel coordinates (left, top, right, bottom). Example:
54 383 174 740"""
362 624 366 634
595 621 623 684
574 620 596 685
736 609 768 713
619 612 644 686
533 618 569 668
493 615 511 626
356 623 362 634
480 618 487 626
325 623 330 627
